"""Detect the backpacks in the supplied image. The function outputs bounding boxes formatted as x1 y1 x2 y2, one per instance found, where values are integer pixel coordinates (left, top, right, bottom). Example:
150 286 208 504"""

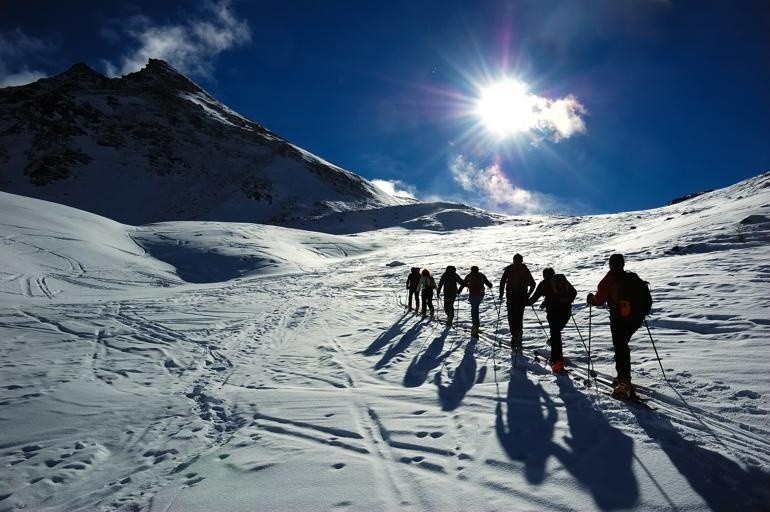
617 270 653 319
546 274 570 303
424 276 436 289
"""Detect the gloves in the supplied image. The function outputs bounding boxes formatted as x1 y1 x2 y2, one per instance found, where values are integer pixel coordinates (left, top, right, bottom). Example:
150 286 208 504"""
587 293 593 304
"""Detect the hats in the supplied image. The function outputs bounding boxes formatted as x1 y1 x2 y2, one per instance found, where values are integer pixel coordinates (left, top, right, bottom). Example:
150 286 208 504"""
609 253 625 267
543 268 555 278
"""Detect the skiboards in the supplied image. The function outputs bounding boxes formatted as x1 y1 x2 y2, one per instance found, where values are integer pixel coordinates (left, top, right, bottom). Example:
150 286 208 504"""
533 351 583 386
583 370 658 411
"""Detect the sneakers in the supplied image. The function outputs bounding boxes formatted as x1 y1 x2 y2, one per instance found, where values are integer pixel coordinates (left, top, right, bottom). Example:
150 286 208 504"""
613 380 633 395
614 376 631 386
552 361 563 373
406 306 524 352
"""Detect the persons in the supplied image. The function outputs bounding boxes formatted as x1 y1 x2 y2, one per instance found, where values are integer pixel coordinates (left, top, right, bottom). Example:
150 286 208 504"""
587 253 644 395
415 267 438 318
527 267 578 367
498 252 536 348
437 265 464 323
405 266 422 313
457 266 493 338
